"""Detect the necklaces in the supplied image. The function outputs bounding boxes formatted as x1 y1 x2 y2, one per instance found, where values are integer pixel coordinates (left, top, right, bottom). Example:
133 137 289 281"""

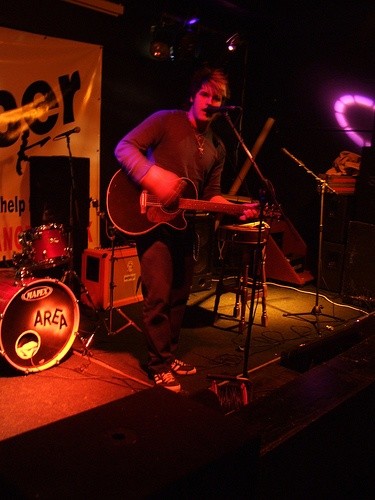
192 128 206 152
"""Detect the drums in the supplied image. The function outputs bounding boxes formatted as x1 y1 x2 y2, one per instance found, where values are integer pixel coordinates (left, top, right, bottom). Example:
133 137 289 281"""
19 222 72 269
214 220 271 245
314 174 357 194
0 270 80 375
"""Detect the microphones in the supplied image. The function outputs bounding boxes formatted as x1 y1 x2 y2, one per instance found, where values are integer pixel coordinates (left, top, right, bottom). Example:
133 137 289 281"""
206 104 238 115
281 147 304 167
53 127 81 141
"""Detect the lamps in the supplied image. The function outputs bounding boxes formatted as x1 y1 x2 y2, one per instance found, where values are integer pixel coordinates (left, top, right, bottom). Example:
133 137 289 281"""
143 20 181 65
225 32 242 54
172 25 204 63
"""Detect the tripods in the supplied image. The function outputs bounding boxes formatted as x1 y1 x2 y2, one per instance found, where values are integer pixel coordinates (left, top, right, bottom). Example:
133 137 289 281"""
82 228 140 350
57 136 102 321
281 165 349 335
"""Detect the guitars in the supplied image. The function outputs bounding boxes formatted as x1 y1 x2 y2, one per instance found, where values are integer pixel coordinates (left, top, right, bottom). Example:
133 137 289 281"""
105 167 284 236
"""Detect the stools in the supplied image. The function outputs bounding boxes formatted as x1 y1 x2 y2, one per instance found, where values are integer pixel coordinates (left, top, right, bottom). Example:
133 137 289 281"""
211 221 270 334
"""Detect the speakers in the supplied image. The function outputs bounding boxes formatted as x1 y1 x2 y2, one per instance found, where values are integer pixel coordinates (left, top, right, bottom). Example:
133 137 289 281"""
82 244 143 311
342 220 375 308
0 381 265 499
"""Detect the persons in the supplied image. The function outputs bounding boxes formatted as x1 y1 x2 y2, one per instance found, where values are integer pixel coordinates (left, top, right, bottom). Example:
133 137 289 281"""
115 66 259 391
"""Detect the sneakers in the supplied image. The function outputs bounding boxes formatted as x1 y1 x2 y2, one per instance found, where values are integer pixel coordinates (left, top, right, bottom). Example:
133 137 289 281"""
170 359 196 374
152 370 180 392
